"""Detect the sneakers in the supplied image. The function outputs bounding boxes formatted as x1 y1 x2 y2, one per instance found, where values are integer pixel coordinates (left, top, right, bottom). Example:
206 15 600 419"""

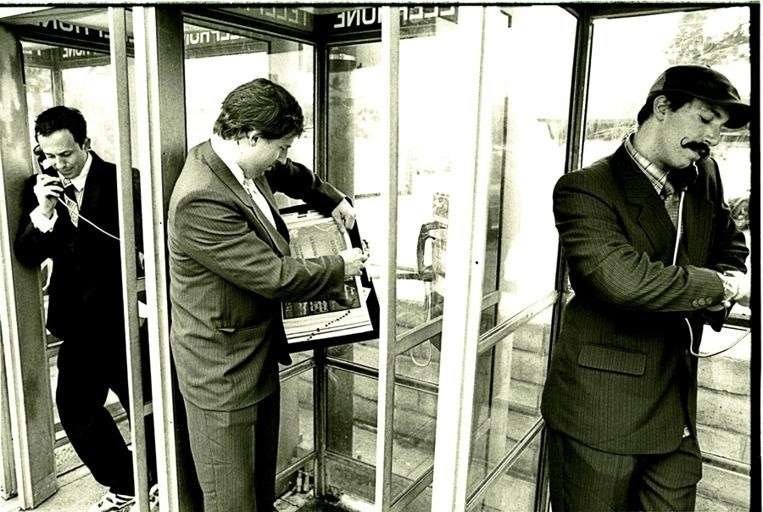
94 484 159 511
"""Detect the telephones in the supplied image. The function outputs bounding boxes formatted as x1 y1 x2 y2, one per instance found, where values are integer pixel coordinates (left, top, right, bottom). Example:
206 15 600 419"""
34 144 60 195
670 160 702 187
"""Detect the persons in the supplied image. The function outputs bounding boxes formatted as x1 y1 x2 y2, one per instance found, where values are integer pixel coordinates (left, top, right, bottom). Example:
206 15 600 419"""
538 65 754 511
163 78 368 510
12 104 158 511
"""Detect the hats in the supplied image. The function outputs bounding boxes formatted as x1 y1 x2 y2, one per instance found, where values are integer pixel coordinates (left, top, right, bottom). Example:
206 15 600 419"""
650 64 751 128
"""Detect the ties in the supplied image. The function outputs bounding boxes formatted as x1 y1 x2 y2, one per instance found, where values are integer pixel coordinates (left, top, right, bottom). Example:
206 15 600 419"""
664 178 683 240
64 187 79 227
